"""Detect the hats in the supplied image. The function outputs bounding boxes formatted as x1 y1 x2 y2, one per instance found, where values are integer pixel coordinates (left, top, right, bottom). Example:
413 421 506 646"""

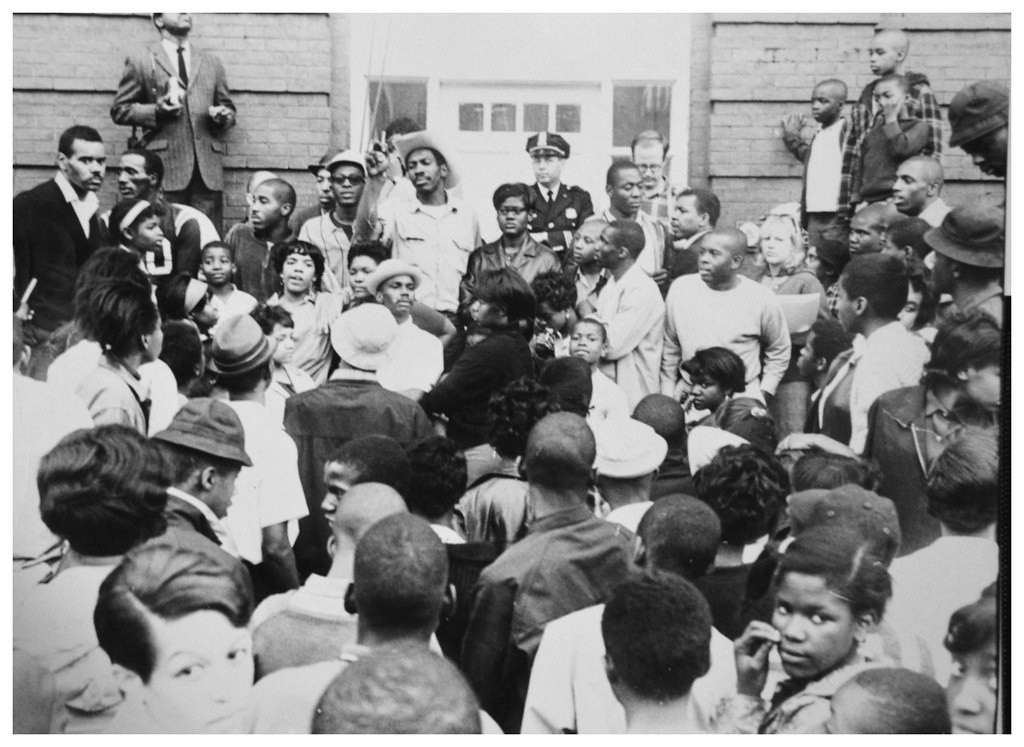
307 149 345 174
111 199 152 235
330 302 408 371
206 312 276 376
366 259 422 298
148 397 254 466
325 147 368 173
922 201 1006 268
592 416 668 479
392 131 461 191
525 131 570 160
947 77 1010 147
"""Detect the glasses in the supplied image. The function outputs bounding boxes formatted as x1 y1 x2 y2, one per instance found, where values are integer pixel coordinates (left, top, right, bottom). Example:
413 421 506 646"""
330 172 366 185
497 205 528 216
757 212 797 238
637 164 663 173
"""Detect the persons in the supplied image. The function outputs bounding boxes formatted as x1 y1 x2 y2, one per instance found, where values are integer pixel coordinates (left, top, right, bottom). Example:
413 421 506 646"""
18 31 1004 679
255 513 503 734
720 526 892 734
827 669 952 734
521 494 778 734
13 421 170 734
943 595 1001 734
94 542 260 734
314 639 484 734
602 570 711 734
110 13 235 237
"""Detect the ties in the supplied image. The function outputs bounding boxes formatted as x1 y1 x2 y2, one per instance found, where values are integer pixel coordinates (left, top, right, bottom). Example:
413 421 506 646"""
177 47 188 89
547 190 553 207
